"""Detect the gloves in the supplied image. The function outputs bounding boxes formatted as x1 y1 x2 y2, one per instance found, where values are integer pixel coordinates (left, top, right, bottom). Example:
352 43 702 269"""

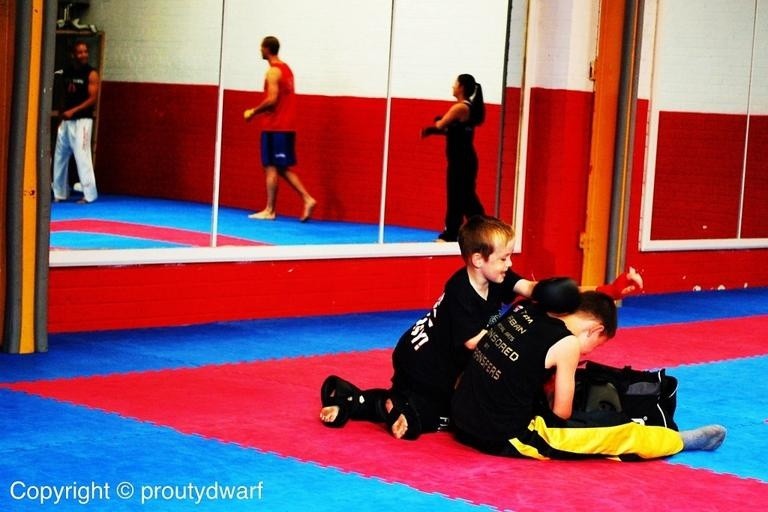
532 275 580 314
576 375 622 416
420 112 448 137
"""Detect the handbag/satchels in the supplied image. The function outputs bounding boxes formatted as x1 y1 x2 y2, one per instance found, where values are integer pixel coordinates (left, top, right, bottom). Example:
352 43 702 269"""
580 359 679 429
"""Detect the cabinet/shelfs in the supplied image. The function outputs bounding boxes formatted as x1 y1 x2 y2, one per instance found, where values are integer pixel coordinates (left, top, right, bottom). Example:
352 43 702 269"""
51 28 105 167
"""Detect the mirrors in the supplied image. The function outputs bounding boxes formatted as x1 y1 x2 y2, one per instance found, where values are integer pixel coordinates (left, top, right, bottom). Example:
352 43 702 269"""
47 0 538 271
638 1 768 253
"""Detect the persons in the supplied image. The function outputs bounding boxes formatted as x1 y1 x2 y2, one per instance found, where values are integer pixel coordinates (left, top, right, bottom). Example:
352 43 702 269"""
446 268 726 461
320 217 539 438
49 42 101 203
422 74 484 242
243 37 316 223
